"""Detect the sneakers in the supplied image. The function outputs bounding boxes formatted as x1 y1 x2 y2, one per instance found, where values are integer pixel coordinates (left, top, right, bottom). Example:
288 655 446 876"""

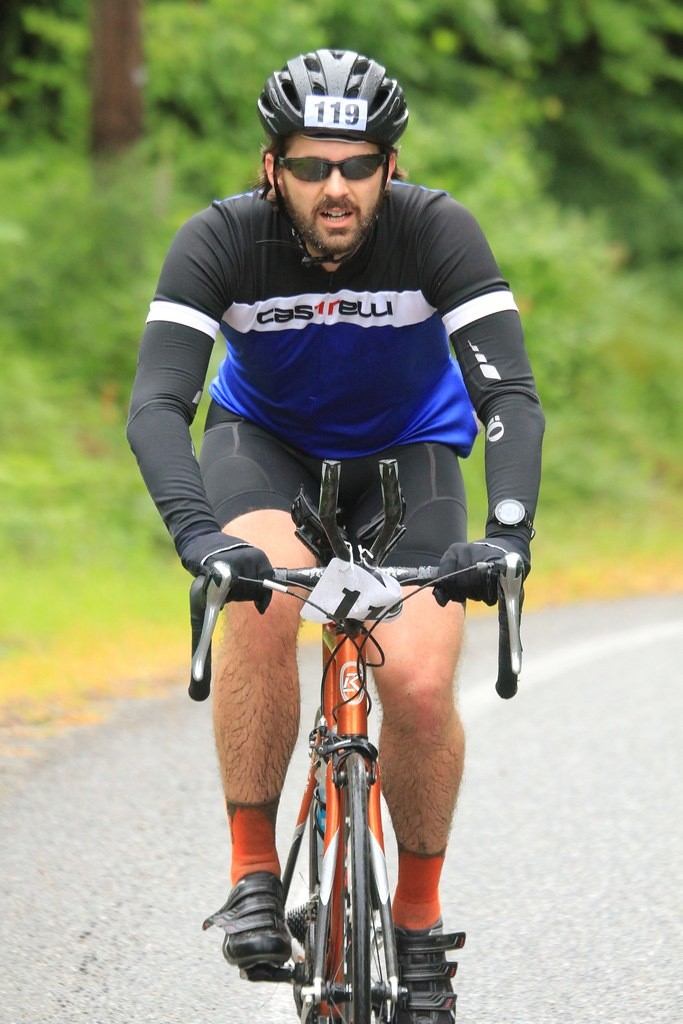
203 872 292 968
395 918 466 1024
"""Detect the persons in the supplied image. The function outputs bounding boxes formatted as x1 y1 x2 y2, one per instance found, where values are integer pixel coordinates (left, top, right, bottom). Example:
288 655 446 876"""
125 49 547 1024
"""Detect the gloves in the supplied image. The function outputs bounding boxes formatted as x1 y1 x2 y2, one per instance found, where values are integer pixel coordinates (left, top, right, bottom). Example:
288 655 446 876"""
433 542 531 607
203 545 275 614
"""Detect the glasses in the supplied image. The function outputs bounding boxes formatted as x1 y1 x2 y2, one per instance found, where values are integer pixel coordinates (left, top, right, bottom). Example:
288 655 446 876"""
277 152 387 183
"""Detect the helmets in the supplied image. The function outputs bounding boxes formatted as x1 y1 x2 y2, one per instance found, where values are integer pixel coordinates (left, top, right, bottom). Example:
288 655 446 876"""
256 49 409 147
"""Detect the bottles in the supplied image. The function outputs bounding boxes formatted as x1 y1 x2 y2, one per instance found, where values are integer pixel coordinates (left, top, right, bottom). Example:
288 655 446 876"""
318 756 327 831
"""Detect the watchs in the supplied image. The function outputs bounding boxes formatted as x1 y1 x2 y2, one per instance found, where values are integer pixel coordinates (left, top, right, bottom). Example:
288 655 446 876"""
486 500 535 540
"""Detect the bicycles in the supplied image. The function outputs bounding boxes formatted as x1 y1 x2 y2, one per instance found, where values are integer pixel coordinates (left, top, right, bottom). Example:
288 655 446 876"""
187 457 525 1023
